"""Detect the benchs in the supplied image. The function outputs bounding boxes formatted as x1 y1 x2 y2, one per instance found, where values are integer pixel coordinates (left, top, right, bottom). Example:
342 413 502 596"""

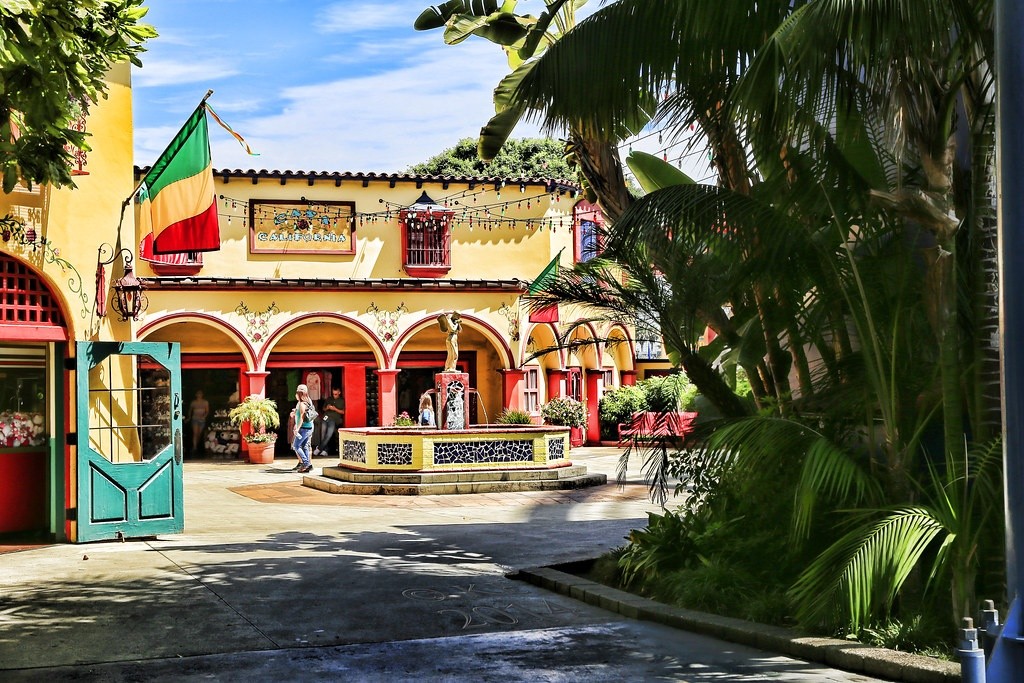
617 411 699 449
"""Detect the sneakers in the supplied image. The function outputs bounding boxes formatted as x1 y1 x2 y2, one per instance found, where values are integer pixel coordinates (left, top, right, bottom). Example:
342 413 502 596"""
320 450 327 456
314 446 320 455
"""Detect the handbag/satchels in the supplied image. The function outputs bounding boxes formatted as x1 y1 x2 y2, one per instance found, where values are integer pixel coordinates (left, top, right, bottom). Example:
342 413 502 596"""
301 403 319 423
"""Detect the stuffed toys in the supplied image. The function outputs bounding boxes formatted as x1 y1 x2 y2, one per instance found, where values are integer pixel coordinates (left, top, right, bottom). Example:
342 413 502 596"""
205 431 240 454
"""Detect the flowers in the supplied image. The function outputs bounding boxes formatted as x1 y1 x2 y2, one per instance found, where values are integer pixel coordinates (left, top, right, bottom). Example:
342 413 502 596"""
243 431 278 444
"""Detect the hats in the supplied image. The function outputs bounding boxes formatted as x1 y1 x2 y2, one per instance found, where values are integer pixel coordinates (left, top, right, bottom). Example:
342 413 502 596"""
297 384 308 393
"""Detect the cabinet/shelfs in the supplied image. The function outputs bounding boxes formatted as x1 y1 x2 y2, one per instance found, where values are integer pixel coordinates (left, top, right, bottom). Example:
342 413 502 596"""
209 417 240 462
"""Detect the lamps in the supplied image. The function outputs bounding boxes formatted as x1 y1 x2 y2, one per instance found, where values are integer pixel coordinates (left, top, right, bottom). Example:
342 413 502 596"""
94 243 149 322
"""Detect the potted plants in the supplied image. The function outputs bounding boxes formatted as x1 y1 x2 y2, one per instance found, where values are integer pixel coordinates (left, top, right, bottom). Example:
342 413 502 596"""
227 394 280 464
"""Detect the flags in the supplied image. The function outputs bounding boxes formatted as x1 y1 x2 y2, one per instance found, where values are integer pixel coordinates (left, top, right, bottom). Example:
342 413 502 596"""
144 105 221 254
529 254 558 323
139 183 187 266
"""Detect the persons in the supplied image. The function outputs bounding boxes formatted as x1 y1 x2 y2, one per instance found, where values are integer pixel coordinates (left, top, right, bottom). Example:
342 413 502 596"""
291 384 315 473
418 394 434 426
313 387 345 457
187 389 210 452
445 310 462 372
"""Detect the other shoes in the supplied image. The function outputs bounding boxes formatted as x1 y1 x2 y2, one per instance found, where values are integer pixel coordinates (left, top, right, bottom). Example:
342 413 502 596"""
298 465 312 472
292 462 303 470
